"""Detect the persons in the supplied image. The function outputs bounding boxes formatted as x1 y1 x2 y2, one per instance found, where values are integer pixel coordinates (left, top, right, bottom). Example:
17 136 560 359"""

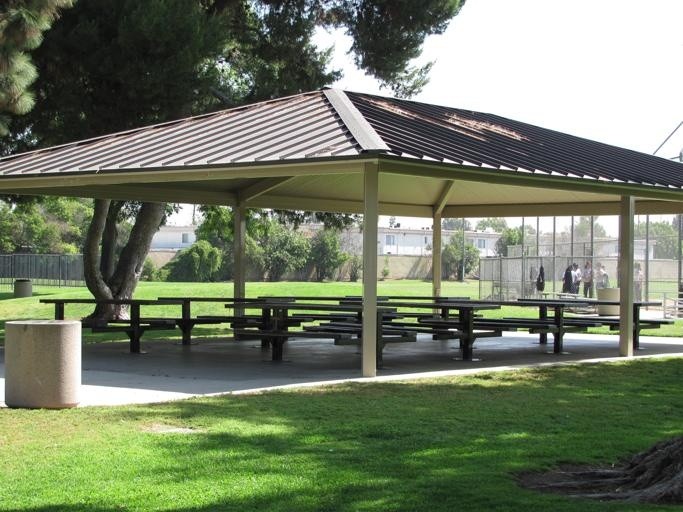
529 264 537 295
560 259 609 299
632 261 644 302
535 266 545 299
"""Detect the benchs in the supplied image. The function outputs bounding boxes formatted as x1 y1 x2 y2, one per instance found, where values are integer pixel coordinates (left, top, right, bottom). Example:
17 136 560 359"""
37 293 678 364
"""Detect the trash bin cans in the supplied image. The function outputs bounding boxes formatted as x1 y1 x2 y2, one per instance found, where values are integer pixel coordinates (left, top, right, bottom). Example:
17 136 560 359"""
2 319 85 408
14 280 32 297
596 286 621 316
